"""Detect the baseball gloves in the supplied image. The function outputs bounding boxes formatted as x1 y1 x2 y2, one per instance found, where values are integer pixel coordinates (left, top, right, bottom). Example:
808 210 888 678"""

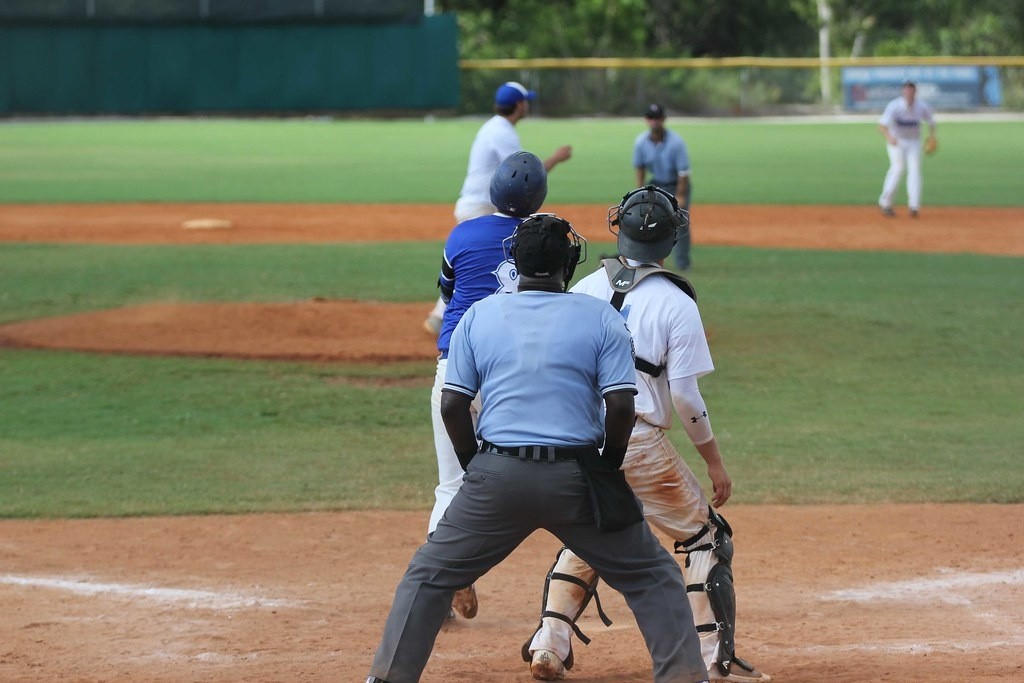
925 137 938 155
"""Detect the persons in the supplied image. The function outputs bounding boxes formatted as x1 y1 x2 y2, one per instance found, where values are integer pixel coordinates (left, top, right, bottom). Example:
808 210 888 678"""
422 81 572 336
878 81 936 218
427 151 548 621
366 212 708 683
523 185 773 683
633 104 691 270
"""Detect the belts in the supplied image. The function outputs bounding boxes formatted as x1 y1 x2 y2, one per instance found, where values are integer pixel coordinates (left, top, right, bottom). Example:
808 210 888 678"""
478 437 580 461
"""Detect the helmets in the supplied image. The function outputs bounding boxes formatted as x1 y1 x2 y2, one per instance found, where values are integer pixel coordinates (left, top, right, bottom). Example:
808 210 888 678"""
489 151 549 216
617 190 679 263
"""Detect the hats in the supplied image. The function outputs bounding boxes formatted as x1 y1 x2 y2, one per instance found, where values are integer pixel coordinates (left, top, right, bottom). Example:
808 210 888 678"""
646 105 664 119
494 81 537 107
512 216 570 277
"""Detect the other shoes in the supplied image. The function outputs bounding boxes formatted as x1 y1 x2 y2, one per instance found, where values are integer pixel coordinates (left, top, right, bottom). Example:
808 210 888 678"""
709 662 771 682
452 586 479 618
530 650 565 680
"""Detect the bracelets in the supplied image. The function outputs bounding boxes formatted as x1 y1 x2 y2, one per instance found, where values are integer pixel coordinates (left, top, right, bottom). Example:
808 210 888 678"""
454 448 477 471
